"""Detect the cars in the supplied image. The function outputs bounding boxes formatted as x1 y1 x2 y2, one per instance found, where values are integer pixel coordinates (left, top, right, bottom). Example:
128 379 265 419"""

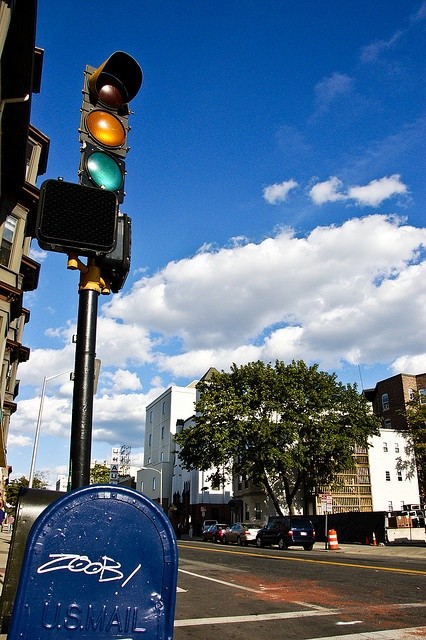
222 523 261 546
200 524 227 543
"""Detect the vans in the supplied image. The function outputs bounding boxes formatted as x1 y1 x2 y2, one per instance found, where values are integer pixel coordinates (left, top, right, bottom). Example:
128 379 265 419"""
201 520 218 531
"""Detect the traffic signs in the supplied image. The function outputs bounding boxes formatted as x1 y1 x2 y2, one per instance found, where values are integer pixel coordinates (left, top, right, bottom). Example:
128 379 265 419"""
321 496 332 511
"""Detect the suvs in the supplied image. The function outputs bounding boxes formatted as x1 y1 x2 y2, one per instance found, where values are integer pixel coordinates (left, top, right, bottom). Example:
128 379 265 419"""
256 516 316 551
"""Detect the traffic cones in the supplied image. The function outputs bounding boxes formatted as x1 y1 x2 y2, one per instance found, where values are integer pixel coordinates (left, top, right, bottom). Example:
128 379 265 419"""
370 532 377 546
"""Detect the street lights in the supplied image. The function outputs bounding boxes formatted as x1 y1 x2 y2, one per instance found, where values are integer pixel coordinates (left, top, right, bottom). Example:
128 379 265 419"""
141 467 162 509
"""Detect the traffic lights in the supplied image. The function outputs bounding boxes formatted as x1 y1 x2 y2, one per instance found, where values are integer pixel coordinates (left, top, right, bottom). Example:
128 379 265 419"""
76 50 143 204
35 182 119 254
113 223 131 292
